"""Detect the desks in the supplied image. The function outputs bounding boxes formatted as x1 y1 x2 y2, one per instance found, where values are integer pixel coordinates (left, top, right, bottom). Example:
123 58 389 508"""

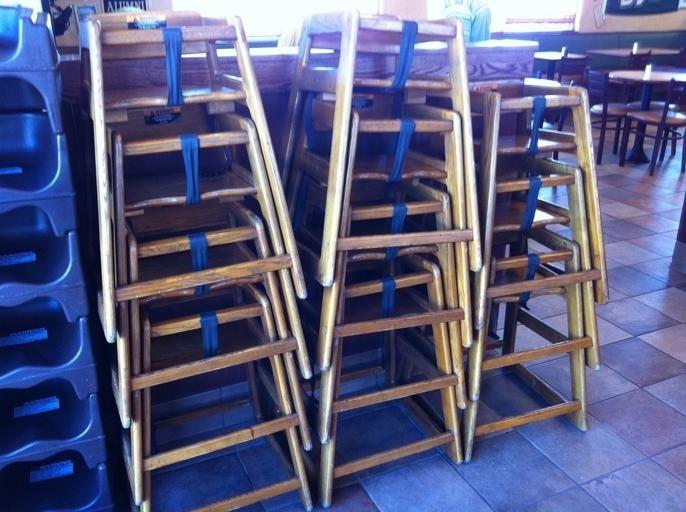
586 46 686 165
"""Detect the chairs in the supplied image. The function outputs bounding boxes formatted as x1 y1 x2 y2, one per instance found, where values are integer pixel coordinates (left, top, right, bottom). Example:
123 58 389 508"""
618 78 686 176
0 1 609 512
